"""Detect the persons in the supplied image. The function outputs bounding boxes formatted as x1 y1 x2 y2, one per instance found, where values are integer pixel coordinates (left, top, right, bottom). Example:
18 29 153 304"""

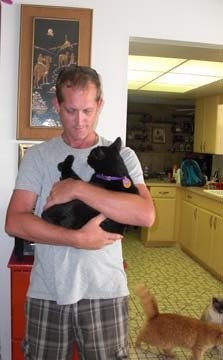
5 64 156 360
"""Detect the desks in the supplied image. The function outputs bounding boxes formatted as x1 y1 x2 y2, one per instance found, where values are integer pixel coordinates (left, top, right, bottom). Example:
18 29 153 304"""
7 246 127 360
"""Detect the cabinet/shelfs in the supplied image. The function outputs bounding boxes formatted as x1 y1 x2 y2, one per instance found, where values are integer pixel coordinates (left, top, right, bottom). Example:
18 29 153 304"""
177 187 200 263
193 95 223 155
141 183 179 247
199 190 223 282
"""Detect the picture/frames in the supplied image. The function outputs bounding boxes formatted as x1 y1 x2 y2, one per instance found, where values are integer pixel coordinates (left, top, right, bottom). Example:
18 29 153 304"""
151 126 165 144
16 4 93 140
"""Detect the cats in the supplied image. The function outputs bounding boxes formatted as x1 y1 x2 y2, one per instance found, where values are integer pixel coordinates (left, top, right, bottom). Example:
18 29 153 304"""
200 296 223 324
42 137 140 236
133 285 223 360
